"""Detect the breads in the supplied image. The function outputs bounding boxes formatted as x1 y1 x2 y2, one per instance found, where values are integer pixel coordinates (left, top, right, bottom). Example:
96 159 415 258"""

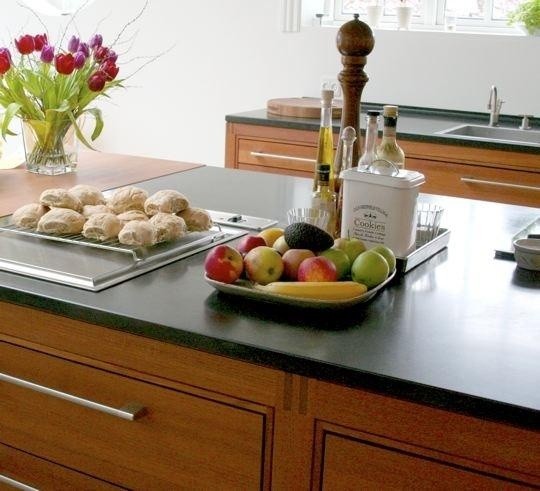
117 221 160 247
143 188 188 215
70 184 106 207
40 189 83 213
37 208 85 236
118 208 147 228
84 203 110 217
11 203 46 229
149 212 183 246
108 187 147 214
83 214 124 241
176 207 212 232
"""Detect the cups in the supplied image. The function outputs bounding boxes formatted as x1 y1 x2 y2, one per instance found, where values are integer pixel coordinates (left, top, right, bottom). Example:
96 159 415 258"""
514 238 540 271
287 207 329 228
415 203 446 246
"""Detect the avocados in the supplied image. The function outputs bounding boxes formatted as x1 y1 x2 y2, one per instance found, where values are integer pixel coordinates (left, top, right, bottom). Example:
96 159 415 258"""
284 222 335 253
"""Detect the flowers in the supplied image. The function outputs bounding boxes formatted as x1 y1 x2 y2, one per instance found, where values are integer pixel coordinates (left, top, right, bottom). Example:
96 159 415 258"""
0 34 127 164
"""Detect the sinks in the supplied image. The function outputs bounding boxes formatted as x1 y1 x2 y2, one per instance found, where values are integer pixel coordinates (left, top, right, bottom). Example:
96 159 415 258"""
433 123 540 147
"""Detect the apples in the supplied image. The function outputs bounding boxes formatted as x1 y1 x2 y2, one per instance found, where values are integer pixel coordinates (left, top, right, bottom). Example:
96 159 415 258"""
205 245 243 283
372 246 396 272
351 250 390 284
318 247 351 277
281 248 315 278
297 256 336 282
244 245 285 284
334 228 366 262
273 234 291 253
257 227 284 245
237 235 266 253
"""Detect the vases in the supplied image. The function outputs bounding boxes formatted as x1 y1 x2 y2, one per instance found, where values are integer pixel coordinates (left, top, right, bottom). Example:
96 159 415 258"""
21 119 79 175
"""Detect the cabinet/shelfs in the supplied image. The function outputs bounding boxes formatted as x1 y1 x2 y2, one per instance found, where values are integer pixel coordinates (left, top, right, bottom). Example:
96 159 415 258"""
225 122 540 207
310 419 540 491
0 332 274 491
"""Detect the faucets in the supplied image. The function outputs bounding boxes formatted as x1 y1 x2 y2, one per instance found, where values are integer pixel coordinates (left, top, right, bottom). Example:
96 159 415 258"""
487 85 505 126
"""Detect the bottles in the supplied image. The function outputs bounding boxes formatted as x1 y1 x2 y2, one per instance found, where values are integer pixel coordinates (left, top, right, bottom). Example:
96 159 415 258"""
338 126 357 220
377 105 406 170
358 109 379 168
310 91 339 236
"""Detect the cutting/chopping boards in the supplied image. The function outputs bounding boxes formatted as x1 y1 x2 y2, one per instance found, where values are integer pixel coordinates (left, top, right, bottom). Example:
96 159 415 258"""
266 98 344 119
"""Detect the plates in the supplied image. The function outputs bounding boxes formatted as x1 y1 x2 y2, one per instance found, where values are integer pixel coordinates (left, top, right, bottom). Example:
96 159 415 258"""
203 269 399 309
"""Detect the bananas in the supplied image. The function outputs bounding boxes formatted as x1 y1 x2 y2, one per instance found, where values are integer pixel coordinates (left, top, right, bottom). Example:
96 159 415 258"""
253 281 366 301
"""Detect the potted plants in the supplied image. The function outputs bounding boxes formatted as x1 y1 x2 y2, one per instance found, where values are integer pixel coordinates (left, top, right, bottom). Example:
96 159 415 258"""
508 0 540 36
